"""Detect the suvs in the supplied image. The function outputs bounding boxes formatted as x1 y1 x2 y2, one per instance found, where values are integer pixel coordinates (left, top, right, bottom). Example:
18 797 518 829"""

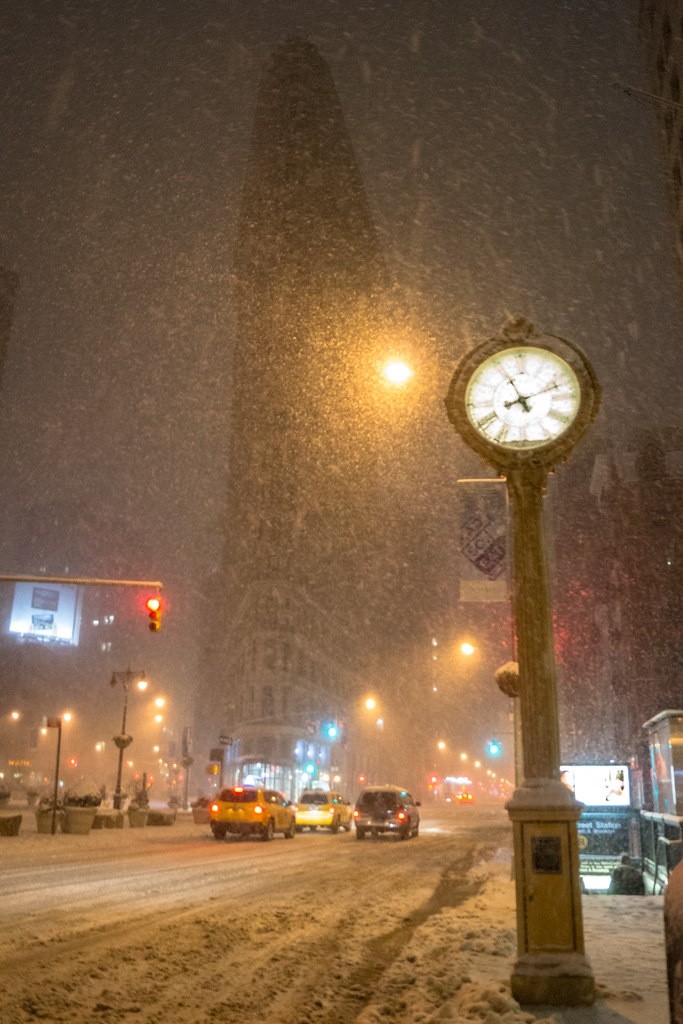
352 786 422 842
209 785 297 841
294 790 352 833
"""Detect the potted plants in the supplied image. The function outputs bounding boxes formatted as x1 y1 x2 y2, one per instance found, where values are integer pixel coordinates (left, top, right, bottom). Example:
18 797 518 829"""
60 782 102 835
127 772 152 828
35 792 65 834
190 795 212 825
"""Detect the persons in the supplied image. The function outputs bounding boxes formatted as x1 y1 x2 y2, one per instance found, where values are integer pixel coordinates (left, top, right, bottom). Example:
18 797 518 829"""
606 856 644 896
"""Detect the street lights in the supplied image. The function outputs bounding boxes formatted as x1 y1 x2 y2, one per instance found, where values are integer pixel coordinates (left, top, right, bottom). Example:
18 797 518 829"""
109 661 147 810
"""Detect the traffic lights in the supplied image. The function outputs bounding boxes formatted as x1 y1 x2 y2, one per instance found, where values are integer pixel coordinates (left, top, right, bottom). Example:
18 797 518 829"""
145 596 160 631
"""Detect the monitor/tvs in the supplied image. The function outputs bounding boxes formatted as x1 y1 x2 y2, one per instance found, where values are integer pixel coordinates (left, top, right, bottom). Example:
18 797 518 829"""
559 764 633 809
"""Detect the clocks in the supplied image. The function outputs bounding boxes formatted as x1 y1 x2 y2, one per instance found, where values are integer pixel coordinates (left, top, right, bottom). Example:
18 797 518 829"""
443 311 602 471
